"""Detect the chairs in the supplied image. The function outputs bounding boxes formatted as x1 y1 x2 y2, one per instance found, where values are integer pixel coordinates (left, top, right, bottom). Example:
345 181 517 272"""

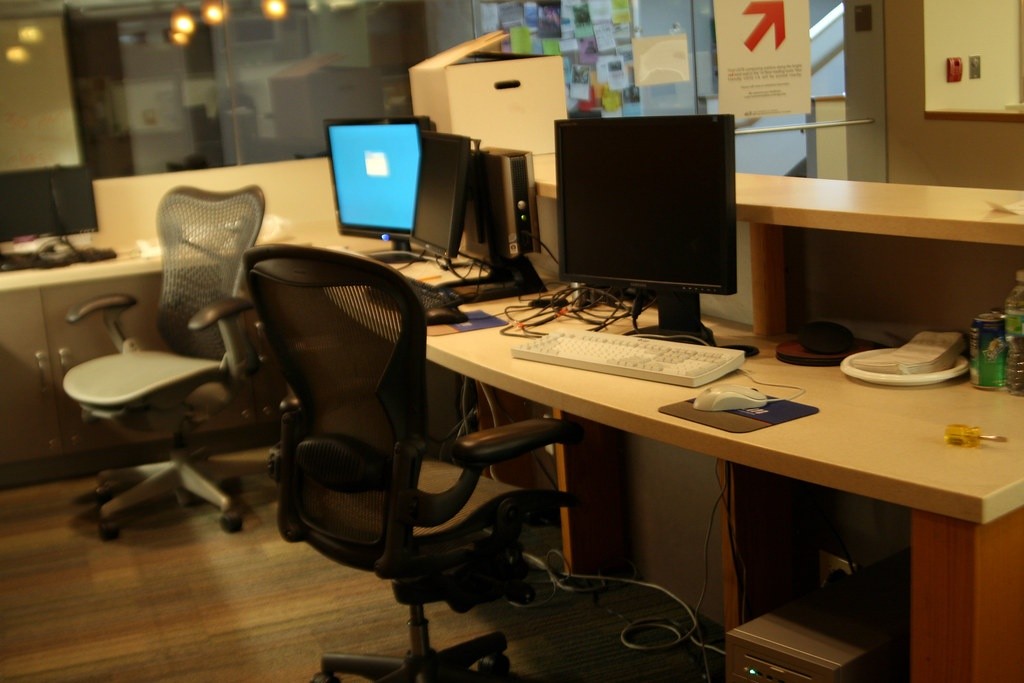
63 186 266 541
244 244 583 683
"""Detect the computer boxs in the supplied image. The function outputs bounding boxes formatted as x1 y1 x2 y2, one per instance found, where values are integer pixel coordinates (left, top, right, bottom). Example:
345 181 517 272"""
726 546 912 683
455 146 541 266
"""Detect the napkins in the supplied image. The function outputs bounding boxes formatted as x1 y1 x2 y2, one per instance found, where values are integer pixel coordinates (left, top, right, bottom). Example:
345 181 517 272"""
854 330 966 377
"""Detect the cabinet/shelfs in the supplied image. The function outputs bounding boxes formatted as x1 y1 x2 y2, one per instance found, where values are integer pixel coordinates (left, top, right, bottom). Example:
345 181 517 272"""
0 256 290 489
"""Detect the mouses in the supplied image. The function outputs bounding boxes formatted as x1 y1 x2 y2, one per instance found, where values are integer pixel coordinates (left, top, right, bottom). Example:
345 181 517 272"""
426 305 469 325
694 382 767 411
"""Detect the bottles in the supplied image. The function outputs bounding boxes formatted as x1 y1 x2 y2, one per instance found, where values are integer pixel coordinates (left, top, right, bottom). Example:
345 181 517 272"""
1005 270 1024 396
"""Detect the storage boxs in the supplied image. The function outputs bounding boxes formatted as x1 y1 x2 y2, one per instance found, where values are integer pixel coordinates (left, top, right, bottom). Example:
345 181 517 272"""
408 30 570 155
270 53 386 154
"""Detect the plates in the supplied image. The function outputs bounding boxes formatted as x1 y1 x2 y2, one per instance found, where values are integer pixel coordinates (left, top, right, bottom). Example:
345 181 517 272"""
839 347 970 386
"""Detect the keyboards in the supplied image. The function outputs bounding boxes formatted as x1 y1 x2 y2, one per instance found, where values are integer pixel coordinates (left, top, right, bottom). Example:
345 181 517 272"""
406 278 466 311
511 327 745 387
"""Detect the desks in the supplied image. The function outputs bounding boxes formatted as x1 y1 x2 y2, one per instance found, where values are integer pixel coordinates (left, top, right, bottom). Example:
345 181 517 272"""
0 149 1024 683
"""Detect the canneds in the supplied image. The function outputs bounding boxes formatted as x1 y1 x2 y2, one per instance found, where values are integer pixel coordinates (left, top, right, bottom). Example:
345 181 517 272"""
971 313 1007 388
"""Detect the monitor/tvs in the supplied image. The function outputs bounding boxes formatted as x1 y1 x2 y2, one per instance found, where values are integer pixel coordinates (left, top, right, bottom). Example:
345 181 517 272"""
554 115 738 346
323 114 431 262
409 131 548 305
0 164 100 262
